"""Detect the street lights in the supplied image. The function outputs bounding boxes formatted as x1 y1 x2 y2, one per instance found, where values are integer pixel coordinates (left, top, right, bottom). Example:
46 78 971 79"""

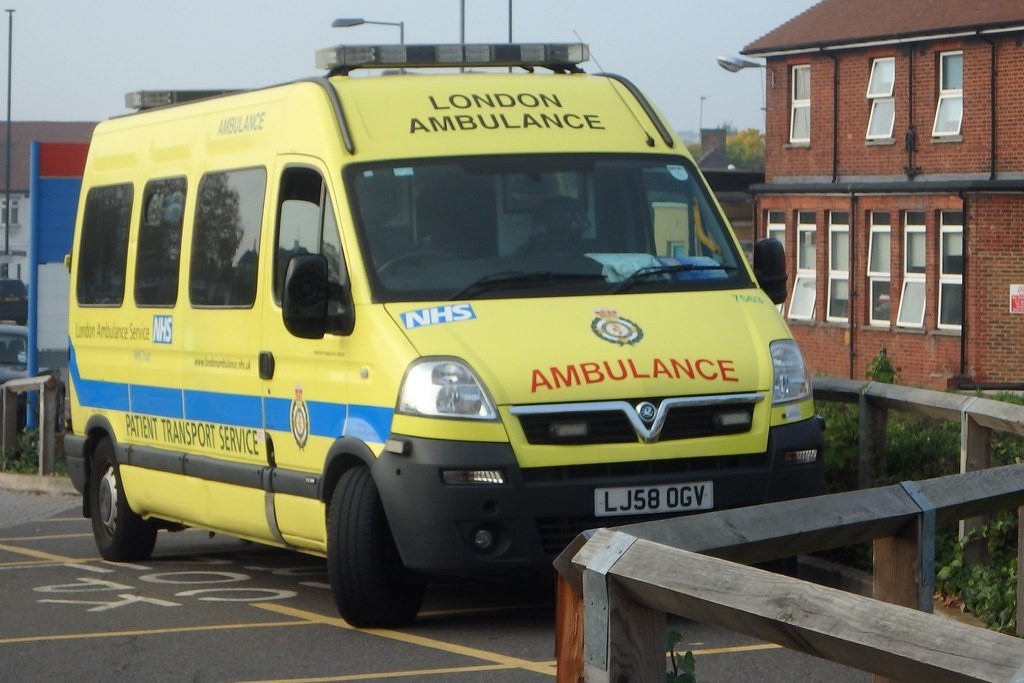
331 18 405 76
5 8 14 278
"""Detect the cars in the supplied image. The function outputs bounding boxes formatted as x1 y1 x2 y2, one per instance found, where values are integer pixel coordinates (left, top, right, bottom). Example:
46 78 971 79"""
0 279 29 326
0 324 56 442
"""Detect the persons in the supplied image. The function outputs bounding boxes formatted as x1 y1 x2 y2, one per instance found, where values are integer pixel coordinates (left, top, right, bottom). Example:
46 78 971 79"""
519 196 601 253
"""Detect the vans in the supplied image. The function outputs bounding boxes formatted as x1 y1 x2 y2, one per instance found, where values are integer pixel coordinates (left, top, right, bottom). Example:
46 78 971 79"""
66 73 831 630
